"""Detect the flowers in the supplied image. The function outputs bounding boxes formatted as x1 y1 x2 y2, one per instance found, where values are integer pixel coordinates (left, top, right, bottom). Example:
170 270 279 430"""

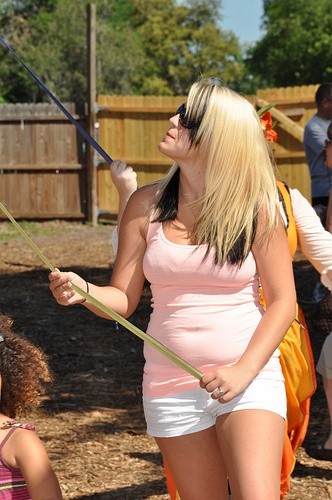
257 103 279 142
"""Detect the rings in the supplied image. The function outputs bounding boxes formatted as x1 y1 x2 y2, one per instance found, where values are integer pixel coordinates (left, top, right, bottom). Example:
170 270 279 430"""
217 389 224 395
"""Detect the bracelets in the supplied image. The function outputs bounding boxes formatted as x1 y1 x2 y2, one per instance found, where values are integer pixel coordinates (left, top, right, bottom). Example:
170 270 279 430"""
80 280 89 303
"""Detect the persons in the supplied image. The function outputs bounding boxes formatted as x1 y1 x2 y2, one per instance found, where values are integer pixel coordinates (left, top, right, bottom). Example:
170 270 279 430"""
48 81 297 500
110 110 332 500
0 316 63 500
303 84 332 232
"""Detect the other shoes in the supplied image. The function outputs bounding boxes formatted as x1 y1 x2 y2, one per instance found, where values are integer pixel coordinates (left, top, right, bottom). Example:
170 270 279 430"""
304 441 332 462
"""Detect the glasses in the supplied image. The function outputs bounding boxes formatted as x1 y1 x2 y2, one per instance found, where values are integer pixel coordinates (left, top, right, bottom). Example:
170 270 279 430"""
174 102 201 130
324 138 332 147
